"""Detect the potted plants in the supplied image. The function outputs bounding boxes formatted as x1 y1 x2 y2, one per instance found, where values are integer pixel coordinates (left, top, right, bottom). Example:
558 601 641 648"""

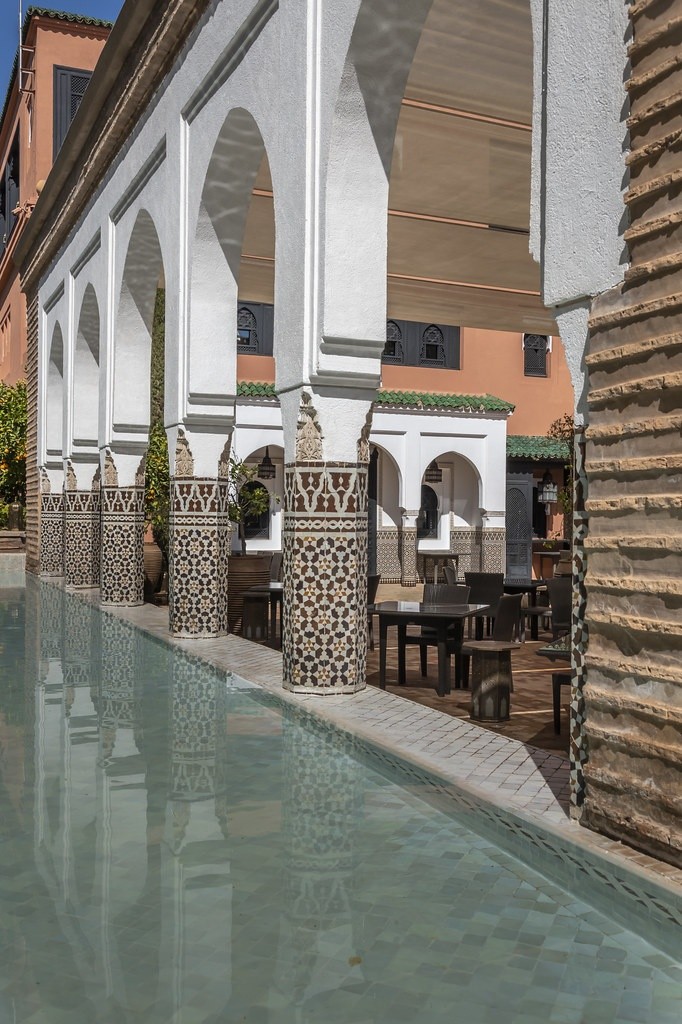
225 448 271 646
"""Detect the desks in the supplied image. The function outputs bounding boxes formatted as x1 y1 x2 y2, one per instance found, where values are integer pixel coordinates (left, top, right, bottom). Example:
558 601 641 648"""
238 590 271 643
504 583 545 640
249 583 284 646
365 600 490 697
535 630 571 737
461 638 520 722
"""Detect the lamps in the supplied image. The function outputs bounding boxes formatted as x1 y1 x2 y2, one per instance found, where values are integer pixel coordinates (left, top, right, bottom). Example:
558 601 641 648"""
258 447 275 479
425 459 442 483
537 468 558 503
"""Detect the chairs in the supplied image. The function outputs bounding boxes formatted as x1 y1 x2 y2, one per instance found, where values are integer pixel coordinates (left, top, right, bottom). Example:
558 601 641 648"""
368 575 381 651
548 577 572 641
464 571 504 642
401 585 471 688
455 593 523 693
